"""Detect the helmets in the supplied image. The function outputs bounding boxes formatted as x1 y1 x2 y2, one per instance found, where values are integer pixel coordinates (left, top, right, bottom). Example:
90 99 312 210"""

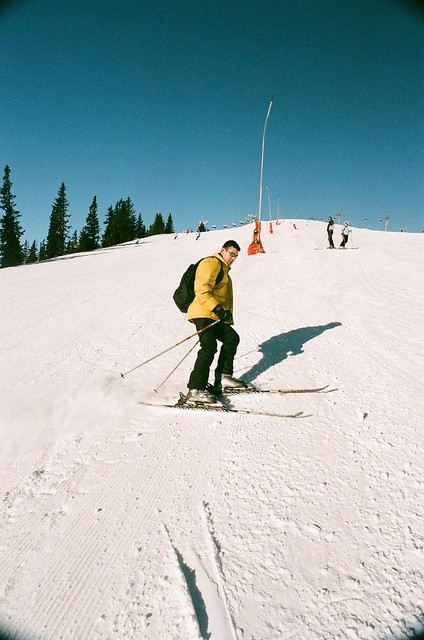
328 216 332 220
344 221 349 226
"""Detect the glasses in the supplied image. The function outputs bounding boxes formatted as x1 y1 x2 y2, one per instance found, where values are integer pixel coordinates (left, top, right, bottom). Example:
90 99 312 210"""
226 248 238 257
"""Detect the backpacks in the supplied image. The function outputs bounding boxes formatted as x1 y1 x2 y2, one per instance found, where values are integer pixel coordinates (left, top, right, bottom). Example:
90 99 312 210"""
173 256 223 313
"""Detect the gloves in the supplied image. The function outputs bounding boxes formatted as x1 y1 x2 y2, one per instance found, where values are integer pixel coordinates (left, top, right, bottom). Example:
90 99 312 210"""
213 305 231 322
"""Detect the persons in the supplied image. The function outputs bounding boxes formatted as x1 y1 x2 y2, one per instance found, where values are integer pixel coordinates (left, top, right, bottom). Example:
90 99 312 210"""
340 221 352 246
326 215 335 249
195 230 200 240
186 240 247 403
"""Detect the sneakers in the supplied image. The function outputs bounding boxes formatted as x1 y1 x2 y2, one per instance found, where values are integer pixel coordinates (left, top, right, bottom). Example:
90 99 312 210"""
214 374 245 388
187 389 216 403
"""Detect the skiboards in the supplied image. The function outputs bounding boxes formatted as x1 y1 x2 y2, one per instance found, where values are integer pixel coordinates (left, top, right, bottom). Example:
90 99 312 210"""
137 384 331 418
